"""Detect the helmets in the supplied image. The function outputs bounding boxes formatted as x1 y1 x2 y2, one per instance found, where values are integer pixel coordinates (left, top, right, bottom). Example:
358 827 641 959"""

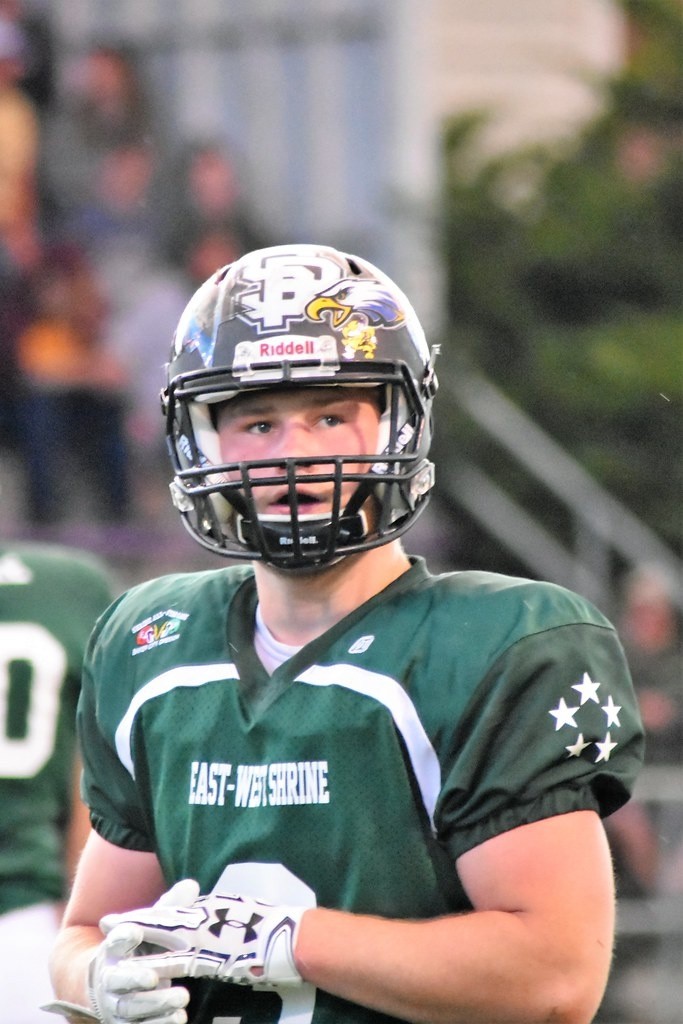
158 244 438 570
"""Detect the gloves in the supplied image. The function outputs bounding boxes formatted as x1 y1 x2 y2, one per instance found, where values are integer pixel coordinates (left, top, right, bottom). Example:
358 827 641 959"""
99 897 303 992
39 879 201 1024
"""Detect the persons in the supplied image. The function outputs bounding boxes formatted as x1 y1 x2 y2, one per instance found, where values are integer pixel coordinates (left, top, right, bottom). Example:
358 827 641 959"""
48 242 647 1024
0 1 283 530
1 439 126 1024
